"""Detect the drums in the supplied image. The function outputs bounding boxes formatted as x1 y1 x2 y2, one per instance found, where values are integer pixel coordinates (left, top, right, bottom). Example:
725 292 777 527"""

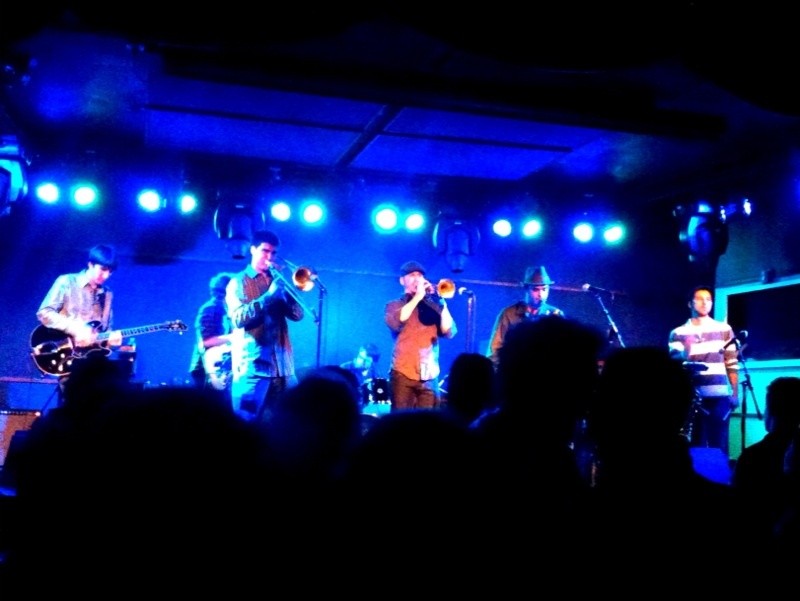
361 378 393 418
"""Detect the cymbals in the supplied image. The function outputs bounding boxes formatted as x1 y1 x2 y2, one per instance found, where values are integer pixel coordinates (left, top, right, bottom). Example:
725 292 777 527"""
681 362 708 372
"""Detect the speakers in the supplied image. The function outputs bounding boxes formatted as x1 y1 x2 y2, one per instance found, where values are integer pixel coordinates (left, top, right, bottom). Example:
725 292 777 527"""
0 412 41 467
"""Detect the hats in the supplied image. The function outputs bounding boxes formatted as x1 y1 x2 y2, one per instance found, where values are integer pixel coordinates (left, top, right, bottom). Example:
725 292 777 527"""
520 266 554 286
399 260 425 276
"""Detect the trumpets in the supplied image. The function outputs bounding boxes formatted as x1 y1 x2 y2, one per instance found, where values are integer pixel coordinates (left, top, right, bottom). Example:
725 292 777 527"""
418 279 456 300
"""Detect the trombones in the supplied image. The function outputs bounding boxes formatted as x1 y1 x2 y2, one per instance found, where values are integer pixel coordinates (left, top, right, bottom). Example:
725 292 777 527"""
265 258 319 322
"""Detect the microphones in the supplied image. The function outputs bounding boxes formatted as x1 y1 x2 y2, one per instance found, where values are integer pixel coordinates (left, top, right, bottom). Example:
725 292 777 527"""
309 274 328 293
582 284 618 296
719 330 748 353
459 287 476 296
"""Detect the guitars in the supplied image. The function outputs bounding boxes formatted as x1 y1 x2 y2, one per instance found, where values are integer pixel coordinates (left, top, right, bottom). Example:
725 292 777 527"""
202 341 234 390
30 320 189 376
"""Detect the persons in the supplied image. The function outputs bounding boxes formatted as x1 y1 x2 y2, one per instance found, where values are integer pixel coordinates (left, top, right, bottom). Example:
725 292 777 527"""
488 266 566 362
0 325 800 601
36 231 459 424
668 285 743 455
